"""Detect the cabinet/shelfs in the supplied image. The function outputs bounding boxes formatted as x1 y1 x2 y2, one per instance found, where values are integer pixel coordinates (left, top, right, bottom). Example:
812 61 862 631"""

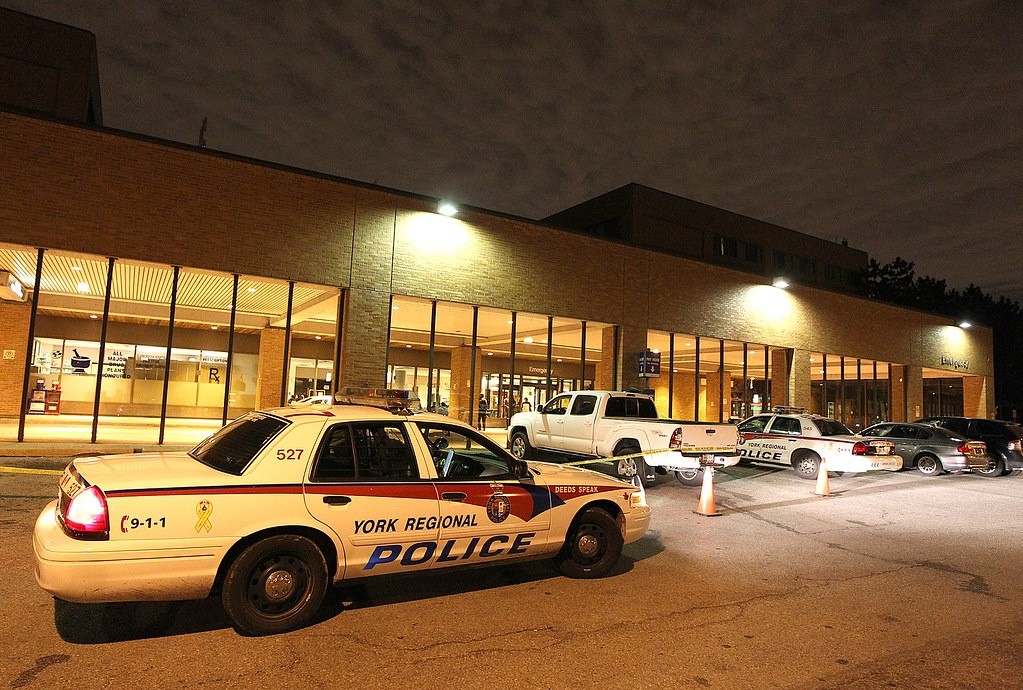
27 390 61 414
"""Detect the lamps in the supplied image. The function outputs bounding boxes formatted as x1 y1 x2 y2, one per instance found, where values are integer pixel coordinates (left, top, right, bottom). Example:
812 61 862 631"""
773 277 790 288
438 199 459 216
957 318 974 329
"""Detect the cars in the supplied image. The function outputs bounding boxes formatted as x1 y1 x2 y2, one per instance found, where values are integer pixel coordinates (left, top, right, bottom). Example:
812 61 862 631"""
30 386 652 638
853 422 991 476
910 415 1023 478
296 393 331 408
735 404 904 480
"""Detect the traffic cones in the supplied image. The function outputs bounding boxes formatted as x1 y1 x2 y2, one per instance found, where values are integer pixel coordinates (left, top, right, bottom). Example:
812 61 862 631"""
693 465 724 516
809 458 834 498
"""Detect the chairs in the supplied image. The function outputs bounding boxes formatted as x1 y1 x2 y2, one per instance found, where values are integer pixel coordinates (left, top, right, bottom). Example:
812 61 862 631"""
377 439 408 476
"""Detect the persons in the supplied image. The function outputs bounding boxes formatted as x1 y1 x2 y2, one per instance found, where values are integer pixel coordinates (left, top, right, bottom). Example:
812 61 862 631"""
287 394 305 404
521 398 532 412
430 402 449 408
478 394 488 432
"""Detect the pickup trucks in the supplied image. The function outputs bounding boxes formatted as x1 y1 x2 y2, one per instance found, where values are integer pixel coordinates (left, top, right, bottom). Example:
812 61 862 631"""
505 389 745 490
